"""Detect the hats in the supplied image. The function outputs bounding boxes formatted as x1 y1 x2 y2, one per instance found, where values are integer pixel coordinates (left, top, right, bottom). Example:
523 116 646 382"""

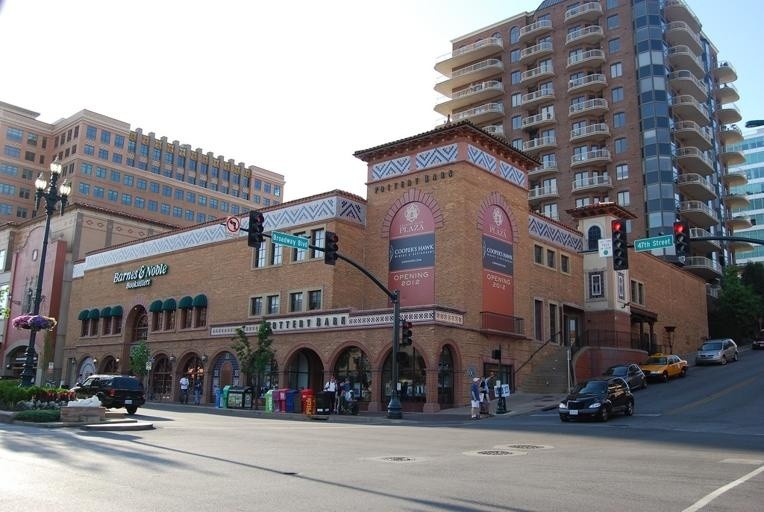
473 378 480 383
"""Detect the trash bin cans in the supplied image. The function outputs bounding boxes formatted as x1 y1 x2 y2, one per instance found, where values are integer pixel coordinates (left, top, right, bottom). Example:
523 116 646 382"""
316 391 331 415
227 386 254 409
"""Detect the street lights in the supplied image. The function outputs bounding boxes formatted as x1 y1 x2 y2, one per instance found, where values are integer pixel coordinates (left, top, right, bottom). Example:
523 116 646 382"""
17 155 71 389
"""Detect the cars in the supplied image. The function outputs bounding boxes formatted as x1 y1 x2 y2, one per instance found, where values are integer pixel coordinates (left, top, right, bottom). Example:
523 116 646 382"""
559 328 764 422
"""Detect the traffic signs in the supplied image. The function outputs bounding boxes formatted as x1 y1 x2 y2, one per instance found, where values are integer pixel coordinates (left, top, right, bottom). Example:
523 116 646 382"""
598 238 612 258
634 235 674 252
271 231 310 251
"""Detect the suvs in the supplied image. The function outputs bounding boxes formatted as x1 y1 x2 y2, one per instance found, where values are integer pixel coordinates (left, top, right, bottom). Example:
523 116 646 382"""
71 375 146 415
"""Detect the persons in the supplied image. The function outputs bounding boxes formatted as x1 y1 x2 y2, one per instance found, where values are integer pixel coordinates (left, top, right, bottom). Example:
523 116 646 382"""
179 374 190 404
339 376 352 397
470 374 496 420
192 376 203 406
323 376 338 413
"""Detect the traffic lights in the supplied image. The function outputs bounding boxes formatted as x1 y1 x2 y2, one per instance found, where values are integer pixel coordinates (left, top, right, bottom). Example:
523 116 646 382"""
248 210 264 248
402 321 412 346
324 231 338 266
492 349 501 360
612 219 629 271
673 220 690 257
